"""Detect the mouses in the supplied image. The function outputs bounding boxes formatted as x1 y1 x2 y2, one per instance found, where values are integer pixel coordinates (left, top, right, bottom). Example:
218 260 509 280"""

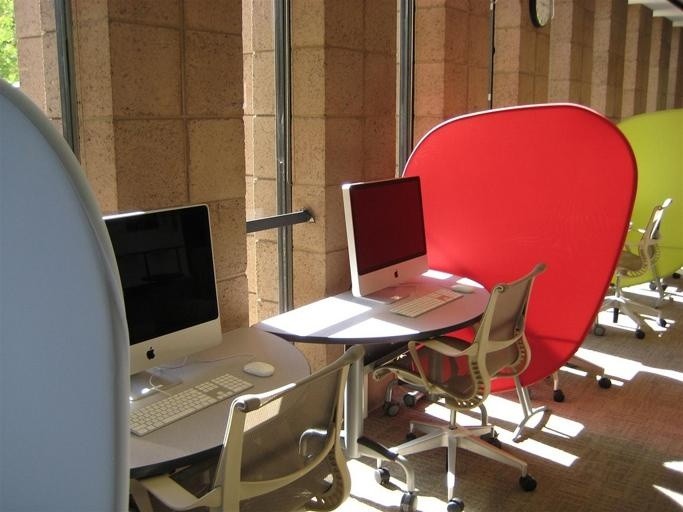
243 361 275 378
451 285 476 294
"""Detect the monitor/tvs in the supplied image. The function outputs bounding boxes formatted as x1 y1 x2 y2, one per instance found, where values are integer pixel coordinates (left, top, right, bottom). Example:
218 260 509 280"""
101 205 223 401
342 175 428 303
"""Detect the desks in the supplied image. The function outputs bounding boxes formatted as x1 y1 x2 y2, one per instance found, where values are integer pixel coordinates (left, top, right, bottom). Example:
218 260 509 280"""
129 326 312 512
249 267 493 511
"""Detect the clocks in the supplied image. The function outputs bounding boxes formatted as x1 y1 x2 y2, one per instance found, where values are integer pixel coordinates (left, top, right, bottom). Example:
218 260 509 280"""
529 0 552 28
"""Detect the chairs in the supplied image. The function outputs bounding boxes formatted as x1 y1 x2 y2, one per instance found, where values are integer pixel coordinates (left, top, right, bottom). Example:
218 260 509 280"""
131 344 365 512
553 355 611 402
593 198 673 339
373 263 547 512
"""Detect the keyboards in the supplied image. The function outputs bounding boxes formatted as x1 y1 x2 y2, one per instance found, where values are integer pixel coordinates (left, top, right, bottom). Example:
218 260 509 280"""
389 287 464 318
128 374 253 437
